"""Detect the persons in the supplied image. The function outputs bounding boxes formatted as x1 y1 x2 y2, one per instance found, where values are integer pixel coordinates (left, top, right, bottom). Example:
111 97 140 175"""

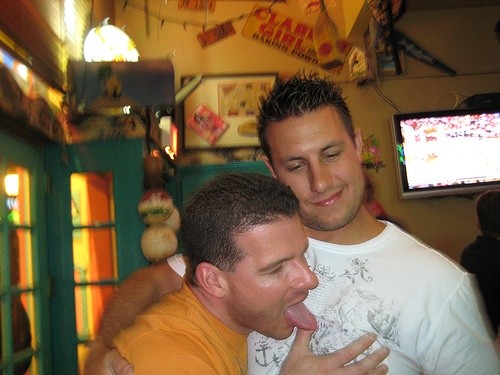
84 68 500 375
112 171 391 375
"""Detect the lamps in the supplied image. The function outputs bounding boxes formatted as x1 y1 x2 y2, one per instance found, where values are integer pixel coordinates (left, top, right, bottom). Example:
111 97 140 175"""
84 24 141 63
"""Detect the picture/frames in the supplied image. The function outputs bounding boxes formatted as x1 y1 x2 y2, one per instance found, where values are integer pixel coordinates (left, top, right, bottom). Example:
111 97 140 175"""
181 74 279 149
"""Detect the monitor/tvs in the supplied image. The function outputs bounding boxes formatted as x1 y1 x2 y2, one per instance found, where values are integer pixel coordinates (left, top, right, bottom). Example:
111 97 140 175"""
391 106 500 200
